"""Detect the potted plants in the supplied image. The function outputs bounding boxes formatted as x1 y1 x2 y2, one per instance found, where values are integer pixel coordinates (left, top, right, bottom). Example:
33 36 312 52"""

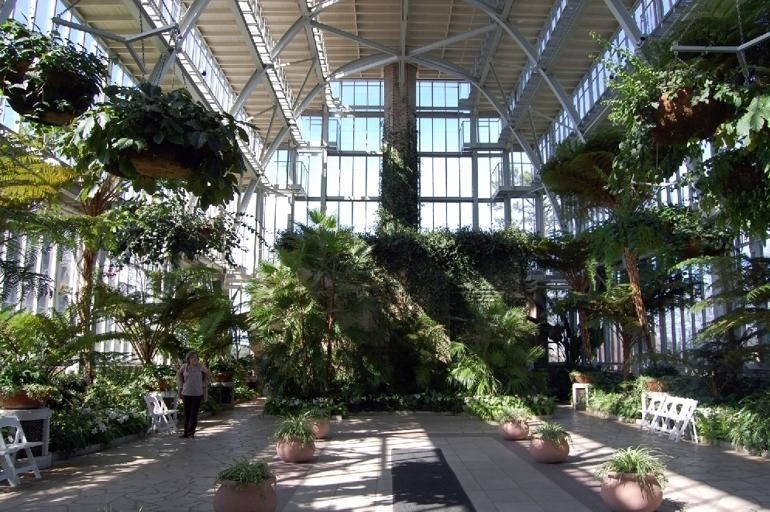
273 405 331 462
65 80 260 213
569 370 591 383
585 30 770 196
591 444 675 512
0 304 96 409
212 455 278 511
0 15 108 130
209 355 237 382
498 405 574 464
636 373 663 392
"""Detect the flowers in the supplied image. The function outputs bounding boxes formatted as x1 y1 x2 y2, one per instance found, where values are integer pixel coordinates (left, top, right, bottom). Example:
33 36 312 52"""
76 373 180 436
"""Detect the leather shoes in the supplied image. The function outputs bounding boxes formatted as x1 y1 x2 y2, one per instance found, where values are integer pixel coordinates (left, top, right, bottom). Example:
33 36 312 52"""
188 434 194 439
179 433 188 438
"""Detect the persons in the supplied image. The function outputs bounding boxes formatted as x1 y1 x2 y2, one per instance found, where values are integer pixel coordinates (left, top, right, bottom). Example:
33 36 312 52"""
174 350 209 441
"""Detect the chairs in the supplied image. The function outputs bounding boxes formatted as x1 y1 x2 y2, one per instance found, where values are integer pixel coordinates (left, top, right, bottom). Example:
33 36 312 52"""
638 394 699 444
0 415 44 488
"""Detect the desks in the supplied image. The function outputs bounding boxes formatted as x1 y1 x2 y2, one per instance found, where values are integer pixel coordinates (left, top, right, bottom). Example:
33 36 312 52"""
573 383 596 411
0 409 55 469
